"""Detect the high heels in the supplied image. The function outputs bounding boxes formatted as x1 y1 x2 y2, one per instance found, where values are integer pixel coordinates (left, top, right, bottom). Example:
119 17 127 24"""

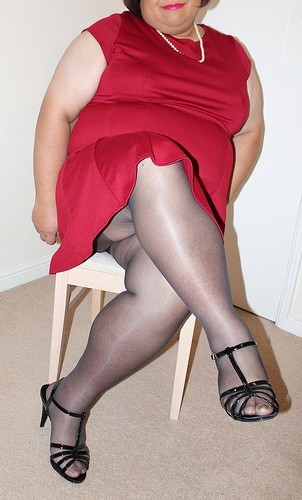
210 337 280 422
39 376 91 485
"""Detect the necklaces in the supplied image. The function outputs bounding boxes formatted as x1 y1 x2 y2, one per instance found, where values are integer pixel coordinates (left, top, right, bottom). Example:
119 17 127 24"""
153 21 206 65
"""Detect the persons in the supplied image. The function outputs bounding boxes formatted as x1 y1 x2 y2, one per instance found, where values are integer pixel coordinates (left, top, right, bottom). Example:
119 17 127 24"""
27 0 285 484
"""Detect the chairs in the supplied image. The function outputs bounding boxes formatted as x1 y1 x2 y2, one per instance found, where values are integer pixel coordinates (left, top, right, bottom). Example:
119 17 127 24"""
47 250 197 421
